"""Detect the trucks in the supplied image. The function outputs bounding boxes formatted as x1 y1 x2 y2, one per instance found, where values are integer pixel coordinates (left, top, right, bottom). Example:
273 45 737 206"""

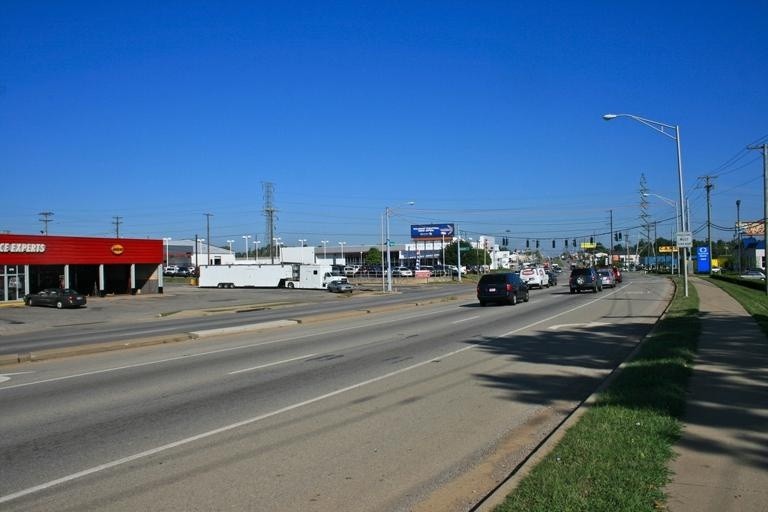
196 263 347 290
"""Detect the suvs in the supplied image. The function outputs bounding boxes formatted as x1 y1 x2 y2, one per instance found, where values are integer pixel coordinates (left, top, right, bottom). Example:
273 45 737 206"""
569 267 603 294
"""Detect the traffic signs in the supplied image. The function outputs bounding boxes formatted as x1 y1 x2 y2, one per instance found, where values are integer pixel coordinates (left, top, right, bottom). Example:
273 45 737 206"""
411 223 454 238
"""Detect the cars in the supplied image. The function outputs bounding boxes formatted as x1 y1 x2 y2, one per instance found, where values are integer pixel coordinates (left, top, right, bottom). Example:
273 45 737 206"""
23 288 87 309
476 272 529 306
515 262 561 289
331 264 491 278
596 266 623 289
163 265 200 277
327 280 355 293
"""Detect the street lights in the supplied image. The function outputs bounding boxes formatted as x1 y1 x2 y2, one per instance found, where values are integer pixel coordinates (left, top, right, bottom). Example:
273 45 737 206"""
602 114 689 298
380 202 414 293
643 192 680 277
735 199 742 275
